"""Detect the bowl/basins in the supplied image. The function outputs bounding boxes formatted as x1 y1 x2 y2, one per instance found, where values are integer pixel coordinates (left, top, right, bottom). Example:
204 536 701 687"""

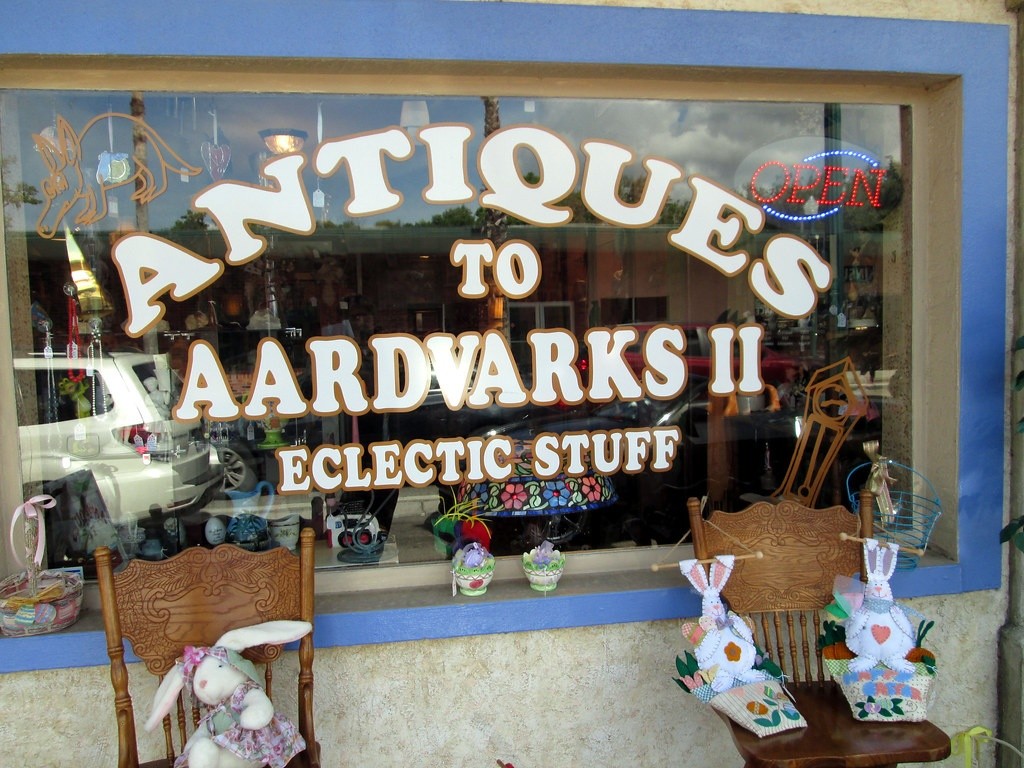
452 570 494 597
523 566 563 591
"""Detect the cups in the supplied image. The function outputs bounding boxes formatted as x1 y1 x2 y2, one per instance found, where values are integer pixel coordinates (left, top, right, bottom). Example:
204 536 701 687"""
271 512 299 551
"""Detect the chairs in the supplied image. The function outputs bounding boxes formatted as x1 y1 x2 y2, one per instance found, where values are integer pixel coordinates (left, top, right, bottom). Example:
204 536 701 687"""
685 496 951 768
93 528 320 768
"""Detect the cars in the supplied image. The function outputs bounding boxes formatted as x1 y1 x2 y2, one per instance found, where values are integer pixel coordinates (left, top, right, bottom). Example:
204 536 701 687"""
569 321 805 391
431 380 885 555
13 351 229 576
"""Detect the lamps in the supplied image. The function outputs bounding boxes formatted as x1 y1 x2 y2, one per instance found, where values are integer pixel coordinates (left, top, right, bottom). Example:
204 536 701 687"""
258 128 308 155
457 440 619 550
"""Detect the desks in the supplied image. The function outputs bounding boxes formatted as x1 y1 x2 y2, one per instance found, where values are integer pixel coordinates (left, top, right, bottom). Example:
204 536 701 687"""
291 534 399 569
